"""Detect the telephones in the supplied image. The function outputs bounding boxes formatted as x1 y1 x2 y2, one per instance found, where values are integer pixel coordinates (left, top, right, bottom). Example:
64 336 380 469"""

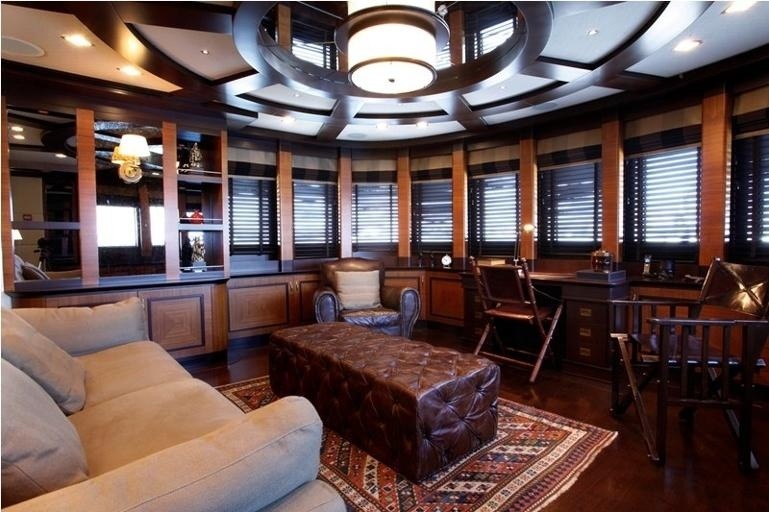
642 254 652 277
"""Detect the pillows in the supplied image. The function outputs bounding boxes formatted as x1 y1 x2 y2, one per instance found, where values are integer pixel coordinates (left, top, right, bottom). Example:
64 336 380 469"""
2 359 92 505
1 307 89 417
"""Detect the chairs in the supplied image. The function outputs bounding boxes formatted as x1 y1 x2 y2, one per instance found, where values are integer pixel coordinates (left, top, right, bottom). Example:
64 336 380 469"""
466 253 569 386
604 254 769 483
311 257 424 341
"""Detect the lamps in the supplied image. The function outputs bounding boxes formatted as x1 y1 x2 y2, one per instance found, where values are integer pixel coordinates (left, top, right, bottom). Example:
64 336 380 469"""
117 132 152 185
110 146 140 166
333 1 452 98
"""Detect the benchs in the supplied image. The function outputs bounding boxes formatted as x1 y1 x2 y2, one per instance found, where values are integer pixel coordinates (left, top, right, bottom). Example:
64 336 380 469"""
266 319 502 487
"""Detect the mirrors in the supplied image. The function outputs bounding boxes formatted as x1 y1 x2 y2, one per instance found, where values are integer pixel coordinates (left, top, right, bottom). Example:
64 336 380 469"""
6 106 83 286
176 138 216 272
93 119 167 277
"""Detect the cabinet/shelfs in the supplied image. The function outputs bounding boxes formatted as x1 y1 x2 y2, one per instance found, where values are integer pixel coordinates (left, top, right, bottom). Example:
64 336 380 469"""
9 279 228 361
2 87 229 295
630 285 769 393
381 266 465 332
222 270 325 339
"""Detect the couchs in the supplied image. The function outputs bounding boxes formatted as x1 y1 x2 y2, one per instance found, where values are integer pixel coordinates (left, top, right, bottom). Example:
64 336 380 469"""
1 292 349 512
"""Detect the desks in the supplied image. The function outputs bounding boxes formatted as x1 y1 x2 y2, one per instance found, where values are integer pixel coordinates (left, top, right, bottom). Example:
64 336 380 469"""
455 268 637 383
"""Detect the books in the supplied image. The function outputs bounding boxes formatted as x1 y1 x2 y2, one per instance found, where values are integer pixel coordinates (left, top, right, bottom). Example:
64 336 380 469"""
22 261 50 280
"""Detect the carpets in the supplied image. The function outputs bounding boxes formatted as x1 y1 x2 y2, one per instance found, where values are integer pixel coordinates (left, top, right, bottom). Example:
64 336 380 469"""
212 372 621 510
